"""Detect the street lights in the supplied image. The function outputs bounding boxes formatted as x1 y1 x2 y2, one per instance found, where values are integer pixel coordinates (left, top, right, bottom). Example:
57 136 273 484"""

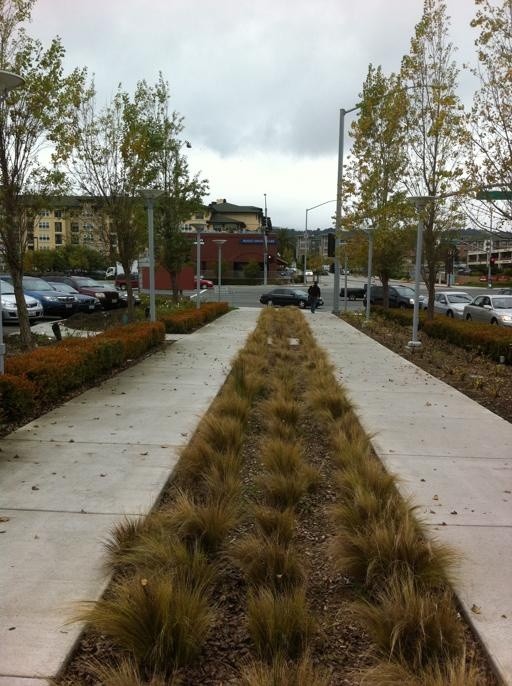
262 192 269 286
331 85 449 315
0 68 26 100
189 220 208 310
360 228 380 327
167 139 193 183
211 239 227 303
136 189 167 322
400 194 441 354
303 198 348 287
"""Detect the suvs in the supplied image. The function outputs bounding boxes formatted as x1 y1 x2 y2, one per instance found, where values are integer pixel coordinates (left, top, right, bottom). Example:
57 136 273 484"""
0 276 81 320
40 276 119 312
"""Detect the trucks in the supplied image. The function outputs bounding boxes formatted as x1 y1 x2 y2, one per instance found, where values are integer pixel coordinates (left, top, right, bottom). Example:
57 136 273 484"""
106 260 139 279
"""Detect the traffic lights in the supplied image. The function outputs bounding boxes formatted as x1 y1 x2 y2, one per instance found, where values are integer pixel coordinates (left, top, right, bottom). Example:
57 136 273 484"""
261 214 267 226
265 226 270 235
266 216 271 227
490 257 495 268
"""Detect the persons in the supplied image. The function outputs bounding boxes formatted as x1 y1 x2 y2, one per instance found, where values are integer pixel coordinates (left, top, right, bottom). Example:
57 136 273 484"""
308 282 320 313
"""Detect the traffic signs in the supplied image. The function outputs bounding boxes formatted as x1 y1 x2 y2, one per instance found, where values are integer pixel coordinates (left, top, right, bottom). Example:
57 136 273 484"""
476 190 512 200
448 239 460 245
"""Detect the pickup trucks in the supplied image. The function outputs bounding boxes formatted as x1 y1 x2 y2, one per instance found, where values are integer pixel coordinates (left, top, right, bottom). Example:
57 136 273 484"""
340 279 384 301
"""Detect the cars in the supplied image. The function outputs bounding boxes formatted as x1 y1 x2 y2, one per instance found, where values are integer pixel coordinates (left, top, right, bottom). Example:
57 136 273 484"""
463 294 512 330
0 279 44 326
422 291 474 321
193 275 213 289
259 288 324 309
496 288 512 295
422 263 512 284
95 281 141 309
46 282 101 316
281 264 350 277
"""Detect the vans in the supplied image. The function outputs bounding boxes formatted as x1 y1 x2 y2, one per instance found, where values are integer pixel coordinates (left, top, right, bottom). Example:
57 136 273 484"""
116 273 140 291
363 284 426 309
